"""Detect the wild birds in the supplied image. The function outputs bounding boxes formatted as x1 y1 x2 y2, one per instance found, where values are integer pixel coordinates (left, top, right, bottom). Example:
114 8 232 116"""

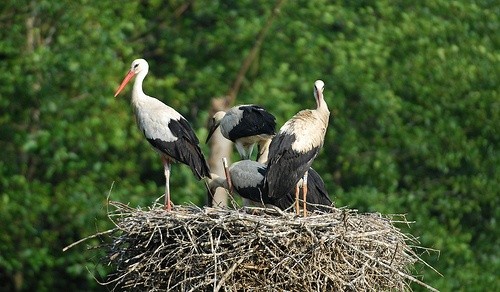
263 79 330 219
205 157 333 217
113 59 212 212
205 103 277 209
